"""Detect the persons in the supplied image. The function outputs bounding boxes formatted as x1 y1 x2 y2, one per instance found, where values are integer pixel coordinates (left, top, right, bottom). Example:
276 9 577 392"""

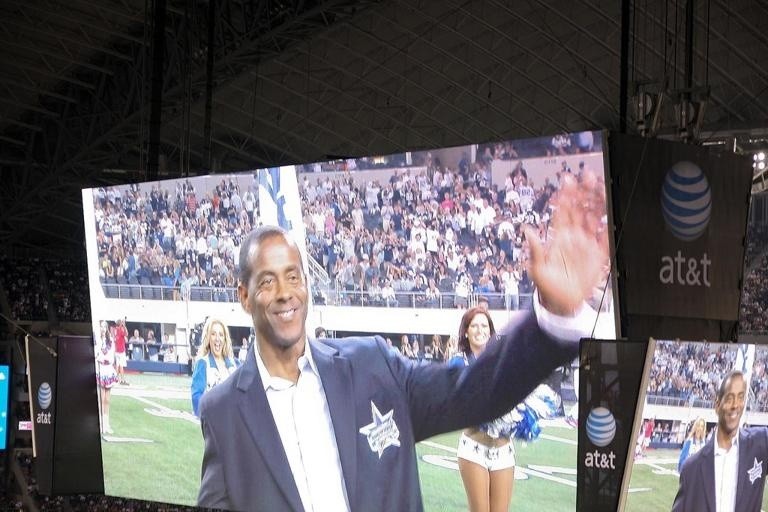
92 130 605 386
2 379 41 511
191 319 239 417
42 495 199 512
94 320 118 434
0 260 91 346
198 171 608 512
740 255 767 335
452 307 515 512
634 341 768 512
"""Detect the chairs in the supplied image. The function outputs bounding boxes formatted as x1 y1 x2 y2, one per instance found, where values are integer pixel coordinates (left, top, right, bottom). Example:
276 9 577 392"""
647 388 704 407
100 212 535 310
